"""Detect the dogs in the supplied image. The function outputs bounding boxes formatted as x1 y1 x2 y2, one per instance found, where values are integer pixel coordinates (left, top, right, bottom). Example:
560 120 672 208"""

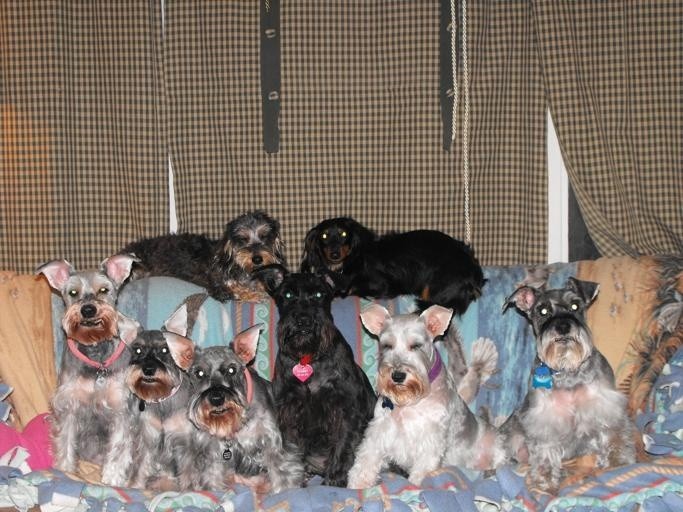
117 210 287 303
249 265 378 488
498 277 645 497
346 303 509 490
300 217 490 322
116 302 193 495
131 323 306 497
34 253 142 489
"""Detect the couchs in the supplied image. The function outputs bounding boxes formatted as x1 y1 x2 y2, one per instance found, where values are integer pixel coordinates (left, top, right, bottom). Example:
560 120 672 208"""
0 254 683 512
